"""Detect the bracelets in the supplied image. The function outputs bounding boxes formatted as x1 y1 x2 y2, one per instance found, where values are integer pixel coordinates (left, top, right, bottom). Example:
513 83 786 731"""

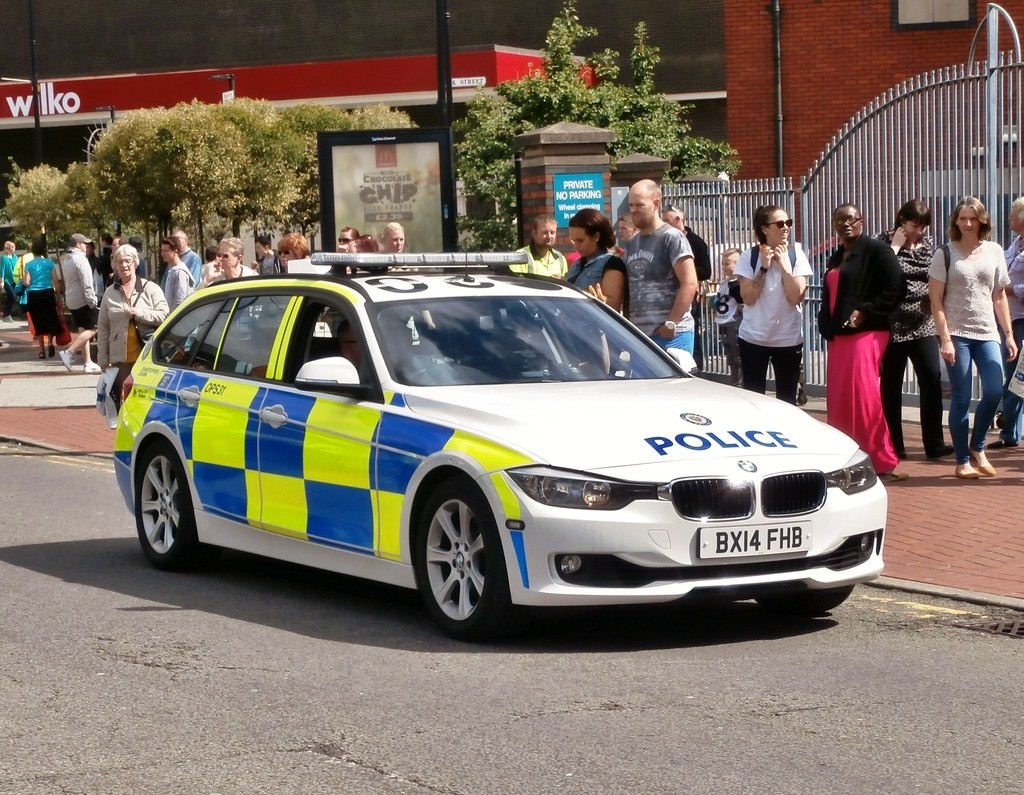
1005 331 1013 335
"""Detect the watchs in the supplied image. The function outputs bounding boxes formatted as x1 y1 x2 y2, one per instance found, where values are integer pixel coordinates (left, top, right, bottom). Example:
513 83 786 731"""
664 321 676 330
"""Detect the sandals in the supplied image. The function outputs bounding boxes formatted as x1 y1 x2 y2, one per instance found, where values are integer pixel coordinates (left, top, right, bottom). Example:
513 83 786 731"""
48 344 55 357
39 352 46 359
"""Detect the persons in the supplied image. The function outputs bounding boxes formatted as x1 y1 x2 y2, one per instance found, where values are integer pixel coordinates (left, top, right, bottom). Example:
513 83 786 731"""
509 215 568 279
161 230 258 314
988 198 1024 448
928 197 1018 478
662 208 712 370
732 206 815 404
97 245 170 415
277 234 310 271
337 319 364 372
566 208 626 313
1 234 146 373
818 204 907 477
872 201 954 457
251 236 283 274
331 222 405 253
618 215 640 240
717 249 742 386
624 179 697 358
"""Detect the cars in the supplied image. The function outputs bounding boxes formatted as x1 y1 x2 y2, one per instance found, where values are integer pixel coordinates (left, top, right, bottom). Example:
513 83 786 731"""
110 250 893 648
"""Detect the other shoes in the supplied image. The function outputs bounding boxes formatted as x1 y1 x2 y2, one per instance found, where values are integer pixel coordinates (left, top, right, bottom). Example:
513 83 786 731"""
879 472 909 482
4 315 15 323
969 451 996 476
954 467 980 479
987 437 1018 449
927 446 955 458
896 449 908 459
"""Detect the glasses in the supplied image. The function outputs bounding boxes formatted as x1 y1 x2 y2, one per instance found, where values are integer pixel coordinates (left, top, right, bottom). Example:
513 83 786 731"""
217 253 235 259
278 250 295 256
339 238 354 245
767 219 792 229
832 218 863 225
661 205 679 215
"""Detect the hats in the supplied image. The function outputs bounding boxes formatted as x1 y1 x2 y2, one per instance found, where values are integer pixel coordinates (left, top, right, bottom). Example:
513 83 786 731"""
70 233 92 246
128 237 142 249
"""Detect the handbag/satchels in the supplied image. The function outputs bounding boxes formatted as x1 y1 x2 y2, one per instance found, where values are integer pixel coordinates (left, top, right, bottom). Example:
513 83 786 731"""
56 302 71 346
920 243 951 315
135 326 158 349
1008 339 1024 398
96 367 119 430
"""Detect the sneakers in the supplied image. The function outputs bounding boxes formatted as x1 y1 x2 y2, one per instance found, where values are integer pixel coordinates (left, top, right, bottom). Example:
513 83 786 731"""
58 351 74 373
84 363 103 374
